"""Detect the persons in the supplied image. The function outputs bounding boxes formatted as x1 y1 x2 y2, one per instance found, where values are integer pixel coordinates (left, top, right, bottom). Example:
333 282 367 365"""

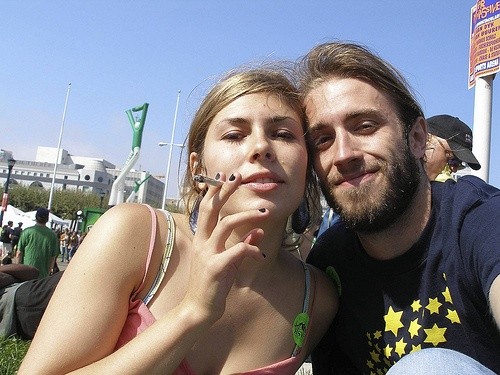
0 208 94 339
298 42 500 375
17 62 341 375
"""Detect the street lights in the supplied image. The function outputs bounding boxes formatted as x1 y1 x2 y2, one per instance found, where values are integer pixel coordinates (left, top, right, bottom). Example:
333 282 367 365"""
0 157 16 228
158 142 186 211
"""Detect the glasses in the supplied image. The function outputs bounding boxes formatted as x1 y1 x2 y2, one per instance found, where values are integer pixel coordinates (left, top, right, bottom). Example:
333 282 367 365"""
432 134 466 170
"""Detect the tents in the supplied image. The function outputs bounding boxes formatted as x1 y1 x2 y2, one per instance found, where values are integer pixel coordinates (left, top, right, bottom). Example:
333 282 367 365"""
1 204 69 233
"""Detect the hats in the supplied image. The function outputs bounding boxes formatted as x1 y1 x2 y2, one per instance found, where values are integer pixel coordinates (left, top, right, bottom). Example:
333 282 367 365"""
37 207 50 218
425 115 481 170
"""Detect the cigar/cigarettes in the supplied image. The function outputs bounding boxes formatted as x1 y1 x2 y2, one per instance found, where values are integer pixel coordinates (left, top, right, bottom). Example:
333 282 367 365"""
191 173 224 189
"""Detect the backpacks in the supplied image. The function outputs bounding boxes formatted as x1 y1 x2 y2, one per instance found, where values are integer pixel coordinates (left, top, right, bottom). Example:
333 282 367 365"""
0 227 12 243
